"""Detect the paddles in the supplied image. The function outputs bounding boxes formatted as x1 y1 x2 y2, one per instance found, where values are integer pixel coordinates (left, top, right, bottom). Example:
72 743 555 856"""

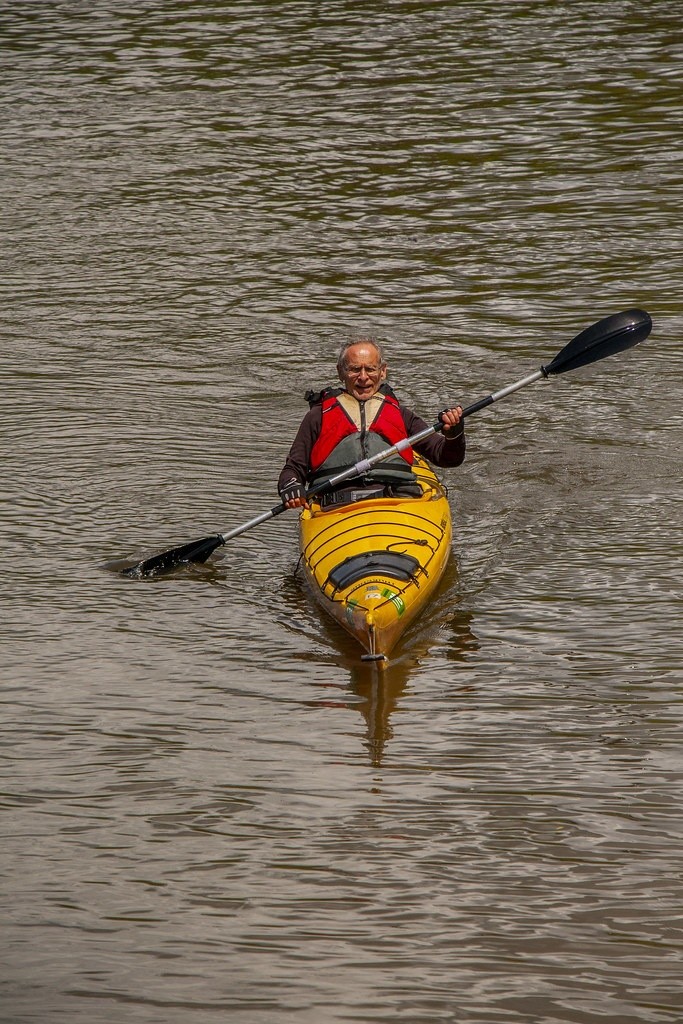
121 308 653 574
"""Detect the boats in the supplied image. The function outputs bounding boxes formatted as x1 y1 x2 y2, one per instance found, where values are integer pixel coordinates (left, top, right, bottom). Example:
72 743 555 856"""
295 447 453 673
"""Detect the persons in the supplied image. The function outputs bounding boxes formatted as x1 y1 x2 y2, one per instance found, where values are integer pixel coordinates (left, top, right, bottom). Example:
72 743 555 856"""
277 339 466 509
